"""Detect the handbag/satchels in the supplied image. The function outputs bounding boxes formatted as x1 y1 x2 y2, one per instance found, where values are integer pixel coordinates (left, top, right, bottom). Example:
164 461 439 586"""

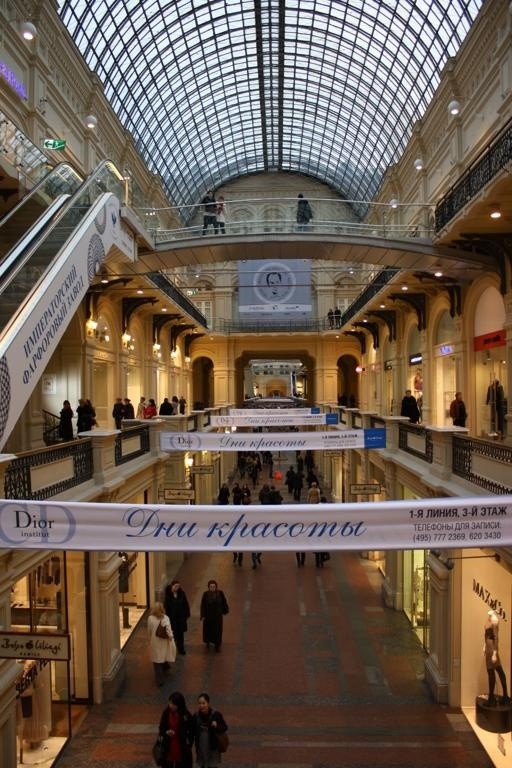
152 735 167 766
155 620 169 639
214 731 230 752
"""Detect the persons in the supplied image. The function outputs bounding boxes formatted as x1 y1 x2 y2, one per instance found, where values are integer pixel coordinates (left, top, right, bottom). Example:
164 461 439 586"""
158 692 194 768
450 392 468 427
218 426 330 570
482 610 510 705
119 552 129 593
192 693 228 768
401 390 420 422
267 271 283 302
328 308 334 330
164 580 190 655
200 580 229 653
294 193 313 231
334 306 341 329
215 196 227 234
59 399 95 451
199 191 218 236
112 395 187 430
486 380 505 441
339 391 356 419
416 392 423 423
147 603 177 685
